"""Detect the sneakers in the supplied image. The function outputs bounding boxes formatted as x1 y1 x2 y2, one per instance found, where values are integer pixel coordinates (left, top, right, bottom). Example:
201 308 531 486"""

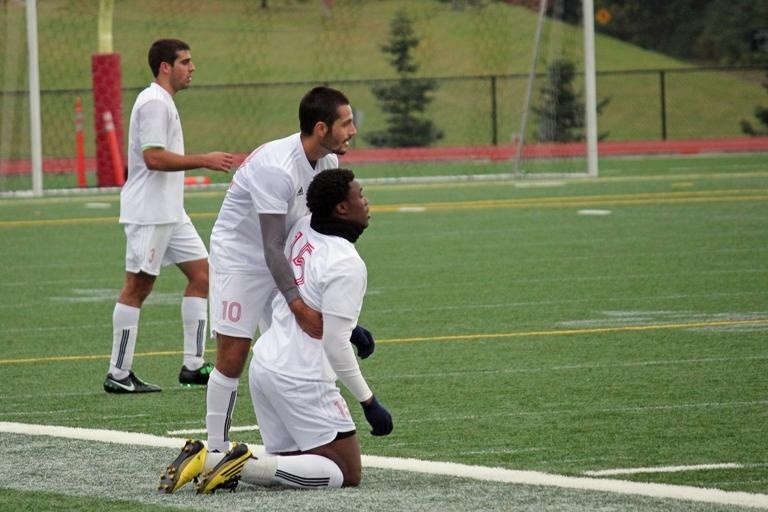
156 440 207 494
178 362 216 387
103 370 163 393
196 442 254 495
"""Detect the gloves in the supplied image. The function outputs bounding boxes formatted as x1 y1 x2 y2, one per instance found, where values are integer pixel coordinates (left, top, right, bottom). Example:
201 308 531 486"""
360 395 393 436
349 325 375 359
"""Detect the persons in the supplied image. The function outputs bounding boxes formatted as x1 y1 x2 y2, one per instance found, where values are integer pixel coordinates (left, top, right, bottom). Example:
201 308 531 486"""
158 167 393 493
202 86 360 446
99 37 234 392
